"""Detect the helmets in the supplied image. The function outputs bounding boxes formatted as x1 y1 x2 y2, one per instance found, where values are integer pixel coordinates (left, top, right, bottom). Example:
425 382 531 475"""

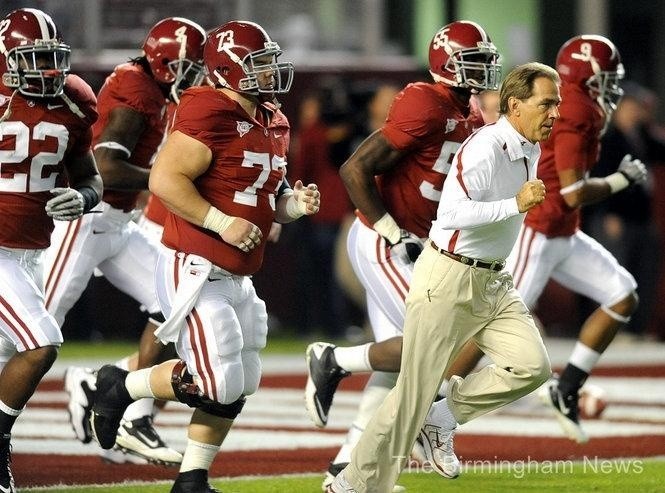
142 7 295 103
556 34 625 122
0 8 72 100
428 21 502 95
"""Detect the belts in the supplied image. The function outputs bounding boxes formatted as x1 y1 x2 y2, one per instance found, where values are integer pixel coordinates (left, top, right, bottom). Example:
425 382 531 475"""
426 241 506 271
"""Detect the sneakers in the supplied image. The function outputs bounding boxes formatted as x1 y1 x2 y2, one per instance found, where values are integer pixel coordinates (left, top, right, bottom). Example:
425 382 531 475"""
0 431 16 493
305 342 460 493
537 379 590 444
63 363 224 493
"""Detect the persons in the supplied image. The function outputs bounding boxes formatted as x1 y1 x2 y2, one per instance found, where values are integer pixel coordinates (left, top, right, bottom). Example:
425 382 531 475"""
0 1 665 493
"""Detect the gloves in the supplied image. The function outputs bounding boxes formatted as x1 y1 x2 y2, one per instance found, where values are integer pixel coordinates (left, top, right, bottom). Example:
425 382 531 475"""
45 187 86 221
390 229 425 265
617 152 648 185
